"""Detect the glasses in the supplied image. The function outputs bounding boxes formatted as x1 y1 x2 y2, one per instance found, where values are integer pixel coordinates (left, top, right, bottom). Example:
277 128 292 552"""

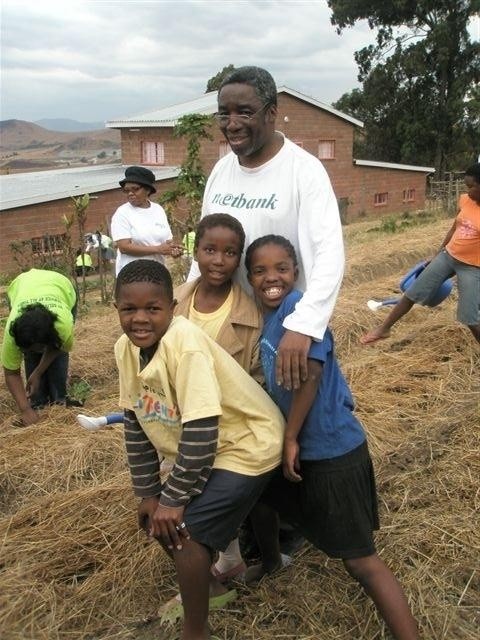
122 186 142 194
214 101 271 128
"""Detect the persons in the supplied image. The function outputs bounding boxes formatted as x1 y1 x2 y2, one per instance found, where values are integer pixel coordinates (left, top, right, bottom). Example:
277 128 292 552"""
185 66 346 559
75 250 92 277
1 268 78 428
84 232 115 266
172 213 265 587
359 162 480 348
110 167 190 277
181 225 196 260
234 233 420 640
111 260 287 640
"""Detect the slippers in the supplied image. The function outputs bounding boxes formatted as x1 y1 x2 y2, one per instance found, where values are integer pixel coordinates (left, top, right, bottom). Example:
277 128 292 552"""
360 331 384 345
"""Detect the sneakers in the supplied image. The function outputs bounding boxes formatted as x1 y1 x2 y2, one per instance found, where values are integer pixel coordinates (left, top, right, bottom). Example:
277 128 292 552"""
156 525 300 631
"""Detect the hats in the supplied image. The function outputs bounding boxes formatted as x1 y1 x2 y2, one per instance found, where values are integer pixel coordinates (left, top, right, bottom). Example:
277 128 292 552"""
118 166 157 196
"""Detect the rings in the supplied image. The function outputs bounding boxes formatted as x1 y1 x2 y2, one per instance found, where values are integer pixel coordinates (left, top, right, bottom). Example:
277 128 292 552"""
176 522 186 531
